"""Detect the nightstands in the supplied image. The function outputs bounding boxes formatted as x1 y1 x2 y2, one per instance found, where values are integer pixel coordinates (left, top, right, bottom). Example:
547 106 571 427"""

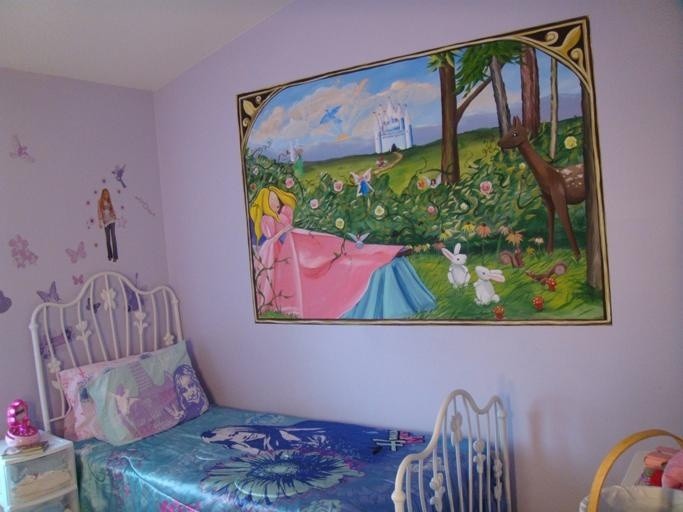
0 429 80 512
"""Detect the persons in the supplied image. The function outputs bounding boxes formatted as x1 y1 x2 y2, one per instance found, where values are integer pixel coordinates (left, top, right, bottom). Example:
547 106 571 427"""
97 188 119 262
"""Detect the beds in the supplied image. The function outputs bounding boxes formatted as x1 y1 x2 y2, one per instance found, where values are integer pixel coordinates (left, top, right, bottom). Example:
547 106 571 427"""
25 271 515 511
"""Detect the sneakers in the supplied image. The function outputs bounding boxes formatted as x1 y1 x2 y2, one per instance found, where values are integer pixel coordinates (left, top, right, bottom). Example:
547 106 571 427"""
108 256 119 263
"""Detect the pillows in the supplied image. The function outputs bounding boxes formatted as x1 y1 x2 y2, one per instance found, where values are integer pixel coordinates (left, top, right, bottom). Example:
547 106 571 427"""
56 352 147 443
74 338 210 448
